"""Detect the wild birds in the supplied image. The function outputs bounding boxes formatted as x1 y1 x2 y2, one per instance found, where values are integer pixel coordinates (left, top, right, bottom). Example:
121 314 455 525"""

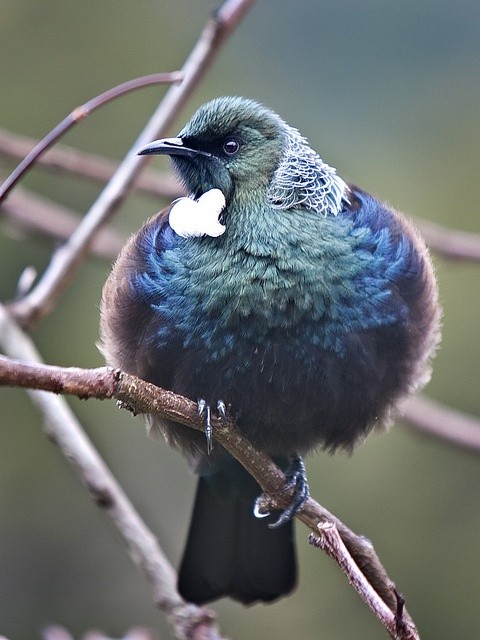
96 96 443 611
